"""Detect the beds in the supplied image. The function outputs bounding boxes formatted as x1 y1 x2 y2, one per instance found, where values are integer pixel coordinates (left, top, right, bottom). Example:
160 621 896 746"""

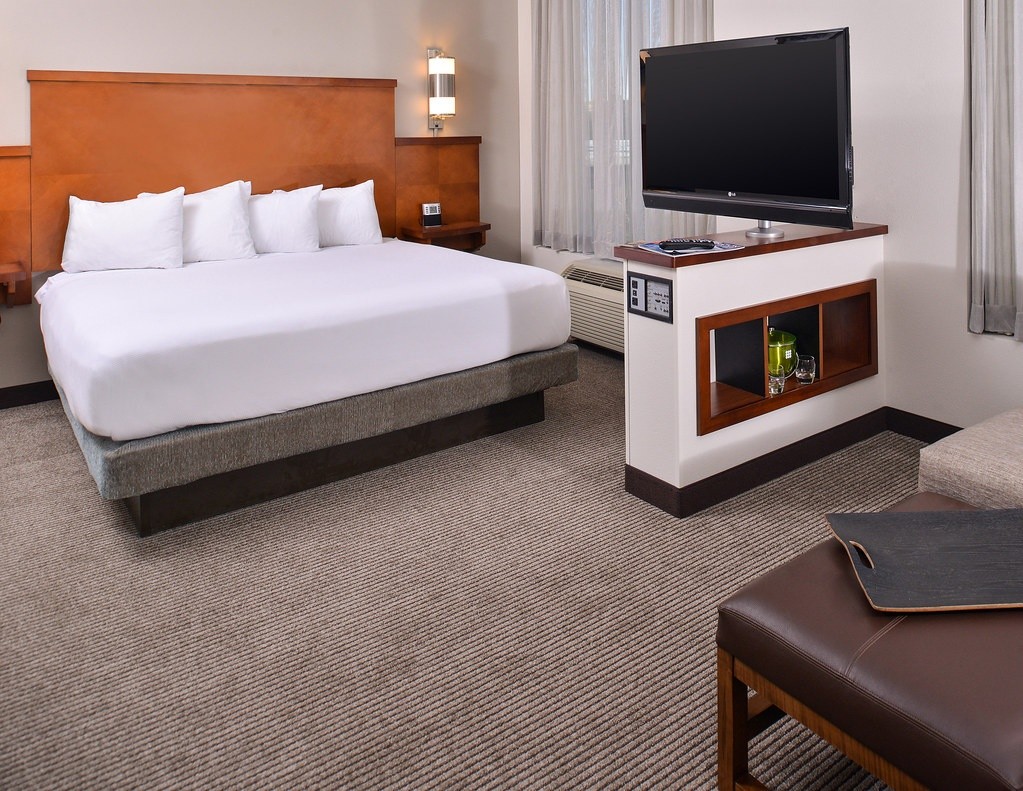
39 237 579 537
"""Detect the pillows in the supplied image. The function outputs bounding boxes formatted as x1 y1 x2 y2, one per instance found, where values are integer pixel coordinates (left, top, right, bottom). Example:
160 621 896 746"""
137 180 258 263
272 180 384 247
59 186 186 272
250 183 325 253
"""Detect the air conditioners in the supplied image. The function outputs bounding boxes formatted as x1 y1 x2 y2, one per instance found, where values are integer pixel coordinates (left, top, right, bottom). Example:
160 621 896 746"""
560 257 624 354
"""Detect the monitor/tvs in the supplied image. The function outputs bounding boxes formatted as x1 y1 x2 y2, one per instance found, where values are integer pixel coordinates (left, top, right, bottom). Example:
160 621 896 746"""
639 26 854 237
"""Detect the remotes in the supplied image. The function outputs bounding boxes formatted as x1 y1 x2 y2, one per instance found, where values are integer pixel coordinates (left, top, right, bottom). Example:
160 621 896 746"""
658 238 715 250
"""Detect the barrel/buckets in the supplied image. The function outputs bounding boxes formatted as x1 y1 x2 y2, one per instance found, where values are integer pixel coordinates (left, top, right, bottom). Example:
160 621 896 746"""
768 330 796 376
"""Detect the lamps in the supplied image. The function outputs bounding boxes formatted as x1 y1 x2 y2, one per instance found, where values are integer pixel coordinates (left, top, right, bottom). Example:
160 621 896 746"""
426 49 457 138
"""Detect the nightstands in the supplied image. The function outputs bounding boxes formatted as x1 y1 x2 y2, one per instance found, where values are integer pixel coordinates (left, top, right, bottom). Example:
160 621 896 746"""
402 222 491 253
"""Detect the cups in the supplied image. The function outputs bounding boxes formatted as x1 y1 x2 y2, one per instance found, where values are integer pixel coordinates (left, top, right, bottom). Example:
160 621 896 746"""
768 364 784 394
796 355 815 384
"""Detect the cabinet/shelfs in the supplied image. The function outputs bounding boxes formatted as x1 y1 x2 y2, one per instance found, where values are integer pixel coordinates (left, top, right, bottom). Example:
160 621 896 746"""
614 221 889 517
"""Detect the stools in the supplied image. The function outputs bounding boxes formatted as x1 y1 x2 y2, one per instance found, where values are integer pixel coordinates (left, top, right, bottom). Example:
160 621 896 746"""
918 405 1023 509
720 490 1023 791
